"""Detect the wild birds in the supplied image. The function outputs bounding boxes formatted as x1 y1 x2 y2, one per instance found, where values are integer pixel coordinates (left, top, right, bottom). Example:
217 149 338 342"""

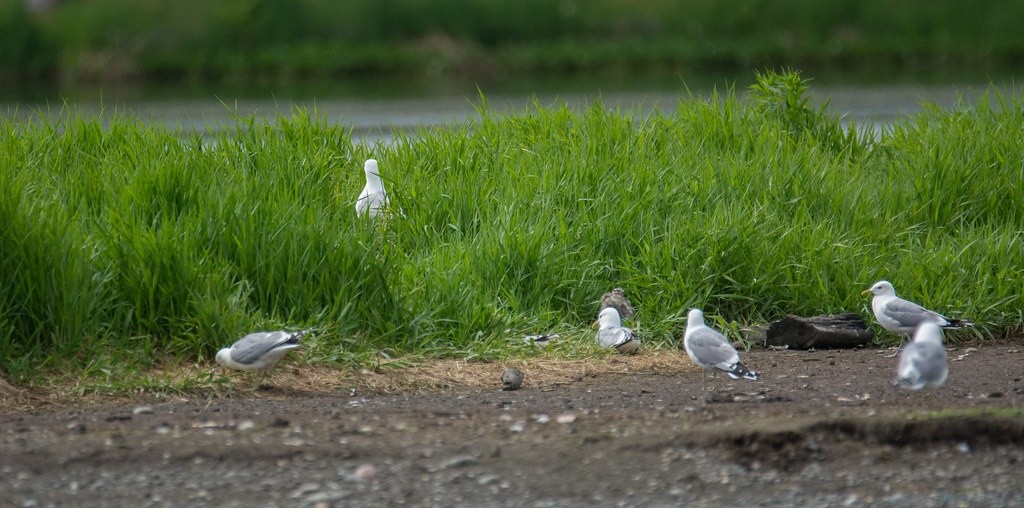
891 321 949 390
683 309 760 391
215 327 316 398
355 159 403 219
860 281 975 358
599 288 634 316
592 307 642 355
